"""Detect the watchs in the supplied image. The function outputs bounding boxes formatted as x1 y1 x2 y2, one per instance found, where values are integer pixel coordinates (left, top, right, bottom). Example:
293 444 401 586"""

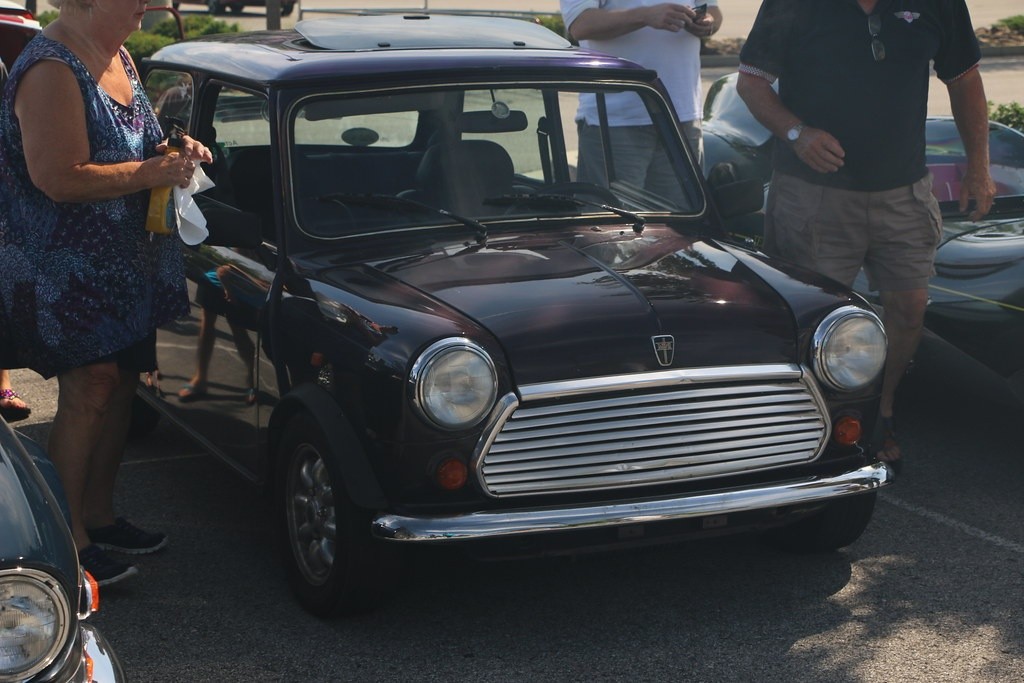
786 122 805 143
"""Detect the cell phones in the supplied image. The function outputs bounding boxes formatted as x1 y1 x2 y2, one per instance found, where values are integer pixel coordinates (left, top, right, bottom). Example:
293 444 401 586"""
683 3 707 29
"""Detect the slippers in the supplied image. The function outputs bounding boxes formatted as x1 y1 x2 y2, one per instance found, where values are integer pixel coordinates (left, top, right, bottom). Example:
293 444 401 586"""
0 389 31 415
244 386 255 403
176 383 208 401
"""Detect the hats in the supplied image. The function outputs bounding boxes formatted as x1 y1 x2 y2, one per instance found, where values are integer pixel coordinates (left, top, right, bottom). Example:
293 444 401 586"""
0 0 42 30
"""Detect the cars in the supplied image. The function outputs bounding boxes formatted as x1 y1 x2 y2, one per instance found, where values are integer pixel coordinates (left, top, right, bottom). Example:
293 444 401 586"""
105 16 897 624
698 68 1024 417
0 417 129 683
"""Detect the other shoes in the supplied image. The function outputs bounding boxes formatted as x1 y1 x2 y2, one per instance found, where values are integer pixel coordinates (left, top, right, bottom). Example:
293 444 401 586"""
867 429 903 485
167 311 197 335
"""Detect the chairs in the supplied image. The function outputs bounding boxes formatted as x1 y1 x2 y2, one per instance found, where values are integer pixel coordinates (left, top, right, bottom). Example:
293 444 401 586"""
398 137 537 209
214 147 275 218
945 179 1015 203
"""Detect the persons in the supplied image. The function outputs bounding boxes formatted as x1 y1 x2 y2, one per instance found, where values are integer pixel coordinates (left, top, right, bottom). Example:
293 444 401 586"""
0 0 215 587
155 73 192 118
179 308 256 403
736 0 998 477
204 262 272 303
559 0 723 211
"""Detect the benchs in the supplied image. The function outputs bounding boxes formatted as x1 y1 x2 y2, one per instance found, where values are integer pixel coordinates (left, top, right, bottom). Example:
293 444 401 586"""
294 150 424 197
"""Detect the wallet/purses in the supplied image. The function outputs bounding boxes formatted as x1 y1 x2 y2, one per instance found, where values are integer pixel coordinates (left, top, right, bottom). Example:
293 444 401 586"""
146 146 188 233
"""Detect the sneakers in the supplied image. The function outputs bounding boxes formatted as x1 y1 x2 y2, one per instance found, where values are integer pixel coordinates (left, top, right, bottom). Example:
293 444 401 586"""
77 544 138 587
84 516 168 554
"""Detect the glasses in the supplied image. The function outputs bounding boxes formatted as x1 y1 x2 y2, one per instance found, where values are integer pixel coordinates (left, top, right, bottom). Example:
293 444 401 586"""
867 12 885 64
